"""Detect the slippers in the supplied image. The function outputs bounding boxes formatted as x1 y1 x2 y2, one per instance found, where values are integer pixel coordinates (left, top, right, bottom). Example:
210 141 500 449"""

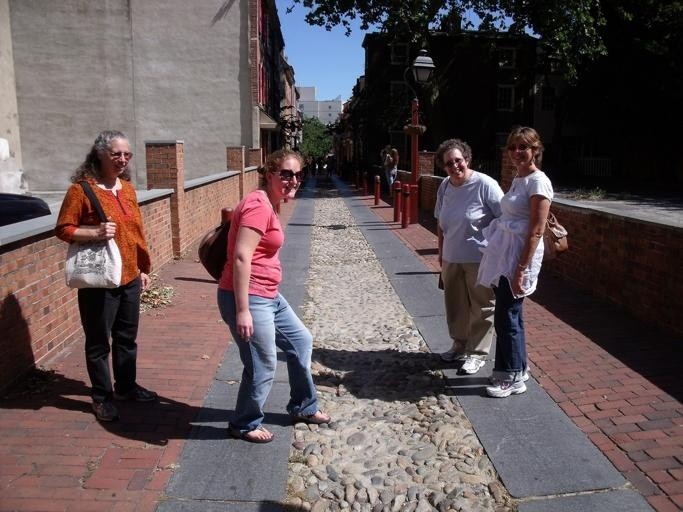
301 408 331 424
228 423 273 443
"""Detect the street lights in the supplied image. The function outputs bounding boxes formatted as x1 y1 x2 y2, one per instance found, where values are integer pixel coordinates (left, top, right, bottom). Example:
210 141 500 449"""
399 48 436 224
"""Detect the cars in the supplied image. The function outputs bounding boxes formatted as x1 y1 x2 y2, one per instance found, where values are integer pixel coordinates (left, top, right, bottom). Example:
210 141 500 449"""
0 192 51 255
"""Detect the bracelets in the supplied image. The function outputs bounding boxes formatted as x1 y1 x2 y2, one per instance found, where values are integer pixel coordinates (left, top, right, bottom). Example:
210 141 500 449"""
517 264 527 270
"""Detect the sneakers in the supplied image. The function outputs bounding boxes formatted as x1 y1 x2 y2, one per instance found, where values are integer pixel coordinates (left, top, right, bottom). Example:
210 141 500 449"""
92 400 121 421
114 385 157 401
440 349 467 362
460 354 485 374
486 370 529 398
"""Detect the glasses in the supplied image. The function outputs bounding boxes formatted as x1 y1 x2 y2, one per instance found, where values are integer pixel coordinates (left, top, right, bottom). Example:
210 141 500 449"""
507 143 529 151
444 157 465 168
271 169 304 182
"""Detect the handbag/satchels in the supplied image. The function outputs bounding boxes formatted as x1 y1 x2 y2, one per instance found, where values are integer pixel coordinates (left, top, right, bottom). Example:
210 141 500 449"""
544 213 568 261
199 220 230 282
63 234 123 290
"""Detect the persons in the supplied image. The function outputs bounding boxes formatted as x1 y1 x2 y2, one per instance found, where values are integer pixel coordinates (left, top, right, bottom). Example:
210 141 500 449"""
55 130 158 423
380 145 399 199
216 149 331 443
434 139 505 374
474 127 554 398
310 157 334 176
